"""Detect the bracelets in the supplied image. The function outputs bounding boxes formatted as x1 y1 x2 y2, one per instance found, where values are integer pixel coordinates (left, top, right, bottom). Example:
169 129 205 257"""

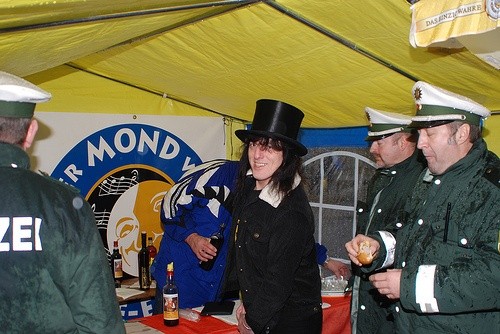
242 321 252 330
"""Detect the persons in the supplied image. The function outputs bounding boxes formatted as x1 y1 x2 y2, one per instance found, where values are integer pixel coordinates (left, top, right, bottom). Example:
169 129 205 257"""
231 98 327 334
346 108 437 334
0 72 127 333
346 81 500 334
149 125 351 311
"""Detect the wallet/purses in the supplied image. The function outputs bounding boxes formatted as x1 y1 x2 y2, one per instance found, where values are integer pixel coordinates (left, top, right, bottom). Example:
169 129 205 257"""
200 301 235 316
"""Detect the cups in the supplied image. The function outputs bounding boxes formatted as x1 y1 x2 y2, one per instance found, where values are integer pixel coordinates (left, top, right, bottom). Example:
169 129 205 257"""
320 274 349 292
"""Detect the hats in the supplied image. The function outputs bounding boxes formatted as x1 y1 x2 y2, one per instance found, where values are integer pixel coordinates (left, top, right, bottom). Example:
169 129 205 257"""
0 71 52 118
235 99 308 157
407 81 491 128
364 106 418 141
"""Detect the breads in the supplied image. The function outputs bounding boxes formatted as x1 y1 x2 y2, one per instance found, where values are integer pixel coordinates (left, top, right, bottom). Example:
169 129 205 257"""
357 240 373 264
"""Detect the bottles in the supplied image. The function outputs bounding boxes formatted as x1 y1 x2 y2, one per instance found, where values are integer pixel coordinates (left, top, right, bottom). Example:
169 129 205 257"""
138 232 150 291
147 237 157 268
111 240 123 288
198 223 228 271
163 264 179 326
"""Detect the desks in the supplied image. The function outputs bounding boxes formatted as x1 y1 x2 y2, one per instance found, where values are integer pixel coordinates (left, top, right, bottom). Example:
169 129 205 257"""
121 287 353 334
115 286 157 305
